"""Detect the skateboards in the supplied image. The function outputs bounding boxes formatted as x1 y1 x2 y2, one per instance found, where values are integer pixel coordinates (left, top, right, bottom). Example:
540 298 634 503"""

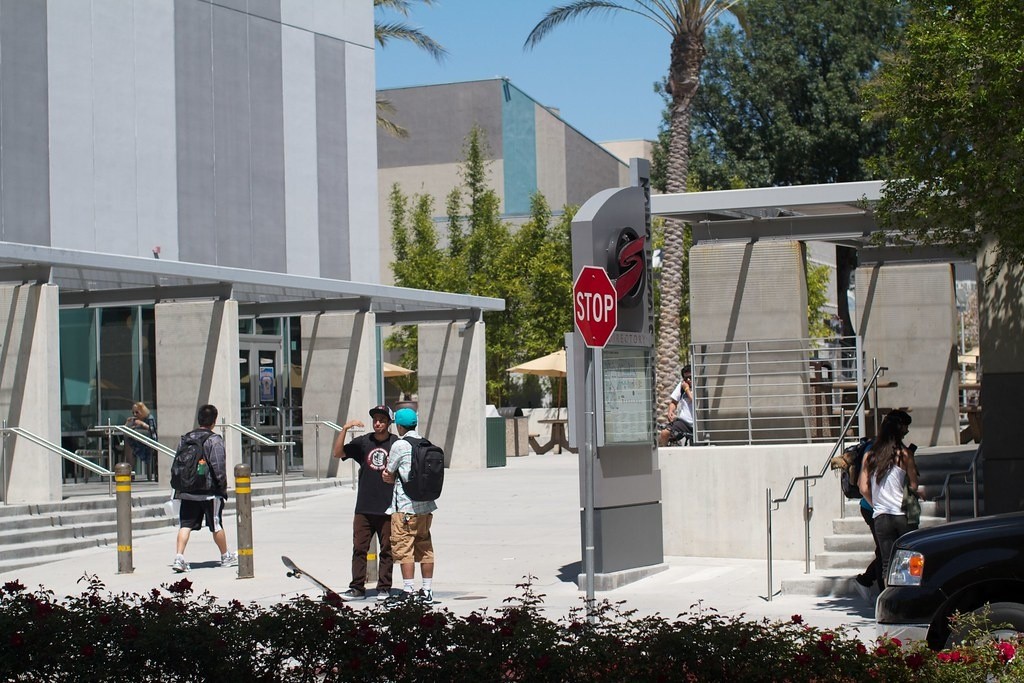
281 555 346 602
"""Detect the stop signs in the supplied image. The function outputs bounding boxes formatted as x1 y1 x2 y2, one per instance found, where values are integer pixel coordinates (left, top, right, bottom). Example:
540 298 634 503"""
574 266 617 349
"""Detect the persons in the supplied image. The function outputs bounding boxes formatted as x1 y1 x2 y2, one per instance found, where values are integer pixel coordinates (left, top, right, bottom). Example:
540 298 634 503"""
659 365 692 447
851 410 926 606
333 405 437 602
124 401 157 471
170 405 238 571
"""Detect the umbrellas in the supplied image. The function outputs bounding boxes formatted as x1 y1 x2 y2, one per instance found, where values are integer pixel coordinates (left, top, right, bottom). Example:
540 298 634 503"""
240 361 302 388
383 362 415 377
505 347 566 420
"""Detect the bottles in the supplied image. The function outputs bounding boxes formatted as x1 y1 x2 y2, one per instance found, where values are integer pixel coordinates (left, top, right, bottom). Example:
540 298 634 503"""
197 459 206 475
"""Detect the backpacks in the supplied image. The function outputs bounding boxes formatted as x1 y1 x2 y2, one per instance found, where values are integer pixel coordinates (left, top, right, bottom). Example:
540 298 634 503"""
838 440 866 498
395 436 444 502
170 431 217 492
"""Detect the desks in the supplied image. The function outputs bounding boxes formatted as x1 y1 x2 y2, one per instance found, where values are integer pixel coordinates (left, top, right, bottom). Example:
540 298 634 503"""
538 420 574 455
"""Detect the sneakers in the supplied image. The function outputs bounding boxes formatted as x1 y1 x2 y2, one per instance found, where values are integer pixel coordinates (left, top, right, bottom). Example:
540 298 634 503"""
338 590 366 601
376 590 389 601
853 578 871 604
221 552 239 568
172 557 192 572
422 589 434 606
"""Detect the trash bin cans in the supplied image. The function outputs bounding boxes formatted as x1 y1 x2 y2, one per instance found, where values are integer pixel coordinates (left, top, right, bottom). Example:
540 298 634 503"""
486 416 507 467
809 361 833 442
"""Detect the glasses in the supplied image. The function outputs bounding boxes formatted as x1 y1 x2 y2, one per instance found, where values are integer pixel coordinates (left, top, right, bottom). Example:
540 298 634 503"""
132 410 139 415
683 375 692 381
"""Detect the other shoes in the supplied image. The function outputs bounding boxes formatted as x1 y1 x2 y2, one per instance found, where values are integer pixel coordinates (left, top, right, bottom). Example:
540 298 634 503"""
386 591 420 606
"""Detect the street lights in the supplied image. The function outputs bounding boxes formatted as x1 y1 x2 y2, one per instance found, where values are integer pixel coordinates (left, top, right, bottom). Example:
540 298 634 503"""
956 287 969 421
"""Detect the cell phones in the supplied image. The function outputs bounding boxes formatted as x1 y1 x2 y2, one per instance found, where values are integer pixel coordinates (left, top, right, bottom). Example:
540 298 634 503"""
909 443 918 453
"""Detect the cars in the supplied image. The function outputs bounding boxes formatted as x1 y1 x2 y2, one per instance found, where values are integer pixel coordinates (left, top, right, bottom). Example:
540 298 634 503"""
874 511 1024 649
100 396 158 426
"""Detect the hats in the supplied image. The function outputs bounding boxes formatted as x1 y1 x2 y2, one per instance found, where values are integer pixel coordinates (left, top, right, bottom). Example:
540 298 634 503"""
369 406 394 422
395 409 419 427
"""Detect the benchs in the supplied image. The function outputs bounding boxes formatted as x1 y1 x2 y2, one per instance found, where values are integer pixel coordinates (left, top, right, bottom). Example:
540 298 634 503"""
528 433 540 455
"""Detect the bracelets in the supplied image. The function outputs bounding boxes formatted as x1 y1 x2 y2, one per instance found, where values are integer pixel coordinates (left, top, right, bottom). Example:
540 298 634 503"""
685 387 689 392
